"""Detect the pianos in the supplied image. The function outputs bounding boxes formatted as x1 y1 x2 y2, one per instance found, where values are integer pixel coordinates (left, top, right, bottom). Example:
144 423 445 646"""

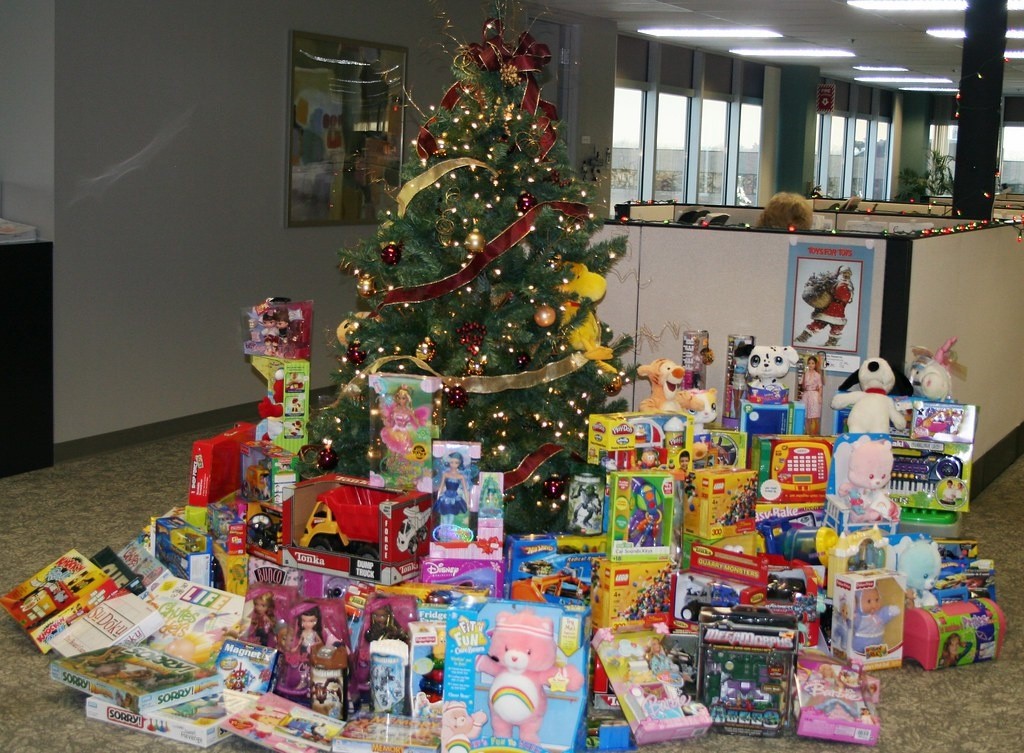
890 452 963 498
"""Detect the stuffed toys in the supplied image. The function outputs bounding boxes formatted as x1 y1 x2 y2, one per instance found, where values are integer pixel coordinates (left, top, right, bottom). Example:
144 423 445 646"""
831 357 914 434
473 610 584 745
734 343 799 389
555 260 619 374
637 358 719 432
838 438 899 523
898 540 941 609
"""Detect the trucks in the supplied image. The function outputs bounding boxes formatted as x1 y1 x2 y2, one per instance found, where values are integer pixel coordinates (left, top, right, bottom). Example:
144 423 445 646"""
299 484 399 562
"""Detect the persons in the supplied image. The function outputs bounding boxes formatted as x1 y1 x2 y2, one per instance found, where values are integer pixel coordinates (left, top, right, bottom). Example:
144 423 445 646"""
678 451 690 472
800 357 822 436
938 633 972 670
838 195 861 211
848 582 901 655
755 191 813 230
940 480 964 506
249 590 408 696
261 311 293 357
830 597 853 651
811 663 860 719
649 637 675 680
379 386 418 463
433 452 469 526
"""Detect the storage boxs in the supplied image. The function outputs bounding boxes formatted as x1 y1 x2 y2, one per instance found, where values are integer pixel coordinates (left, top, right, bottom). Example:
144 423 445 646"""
0 296 980 753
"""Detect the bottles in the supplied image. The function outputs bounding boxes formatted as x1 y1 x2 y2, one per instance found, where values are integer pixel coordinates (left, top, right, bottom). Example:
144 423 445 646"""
310 643 409 720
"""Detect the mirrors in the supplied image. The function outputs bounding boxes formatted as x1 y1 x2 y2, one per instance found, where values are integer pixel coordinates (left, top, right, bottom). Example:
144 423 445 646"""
285 30 407 229
